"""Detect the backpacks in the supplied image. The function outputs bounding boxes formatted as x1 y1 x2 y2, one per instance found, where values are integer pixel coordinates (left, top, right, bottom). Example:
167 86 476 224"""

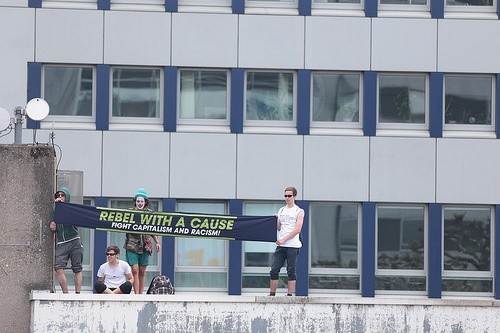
146 275 175 295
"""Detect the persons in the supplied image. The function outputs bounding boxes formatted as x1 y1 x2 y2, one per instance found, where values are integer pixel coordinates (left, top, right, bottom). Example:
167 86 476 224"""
50 186 84 294
270 187 305 296
94 246 133 294
122 189 160 294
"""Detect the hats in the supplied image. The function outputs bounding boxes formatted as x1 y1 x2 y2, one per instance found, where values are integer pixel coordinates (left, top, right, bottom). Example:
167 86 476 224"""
134 188 149 199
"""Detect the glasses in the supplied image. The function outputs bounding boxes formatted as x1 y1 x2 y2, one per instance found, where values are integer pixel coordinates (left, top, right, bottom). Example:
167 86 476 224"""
284 194 294 197
106 253 116 256
56 193 65 196
